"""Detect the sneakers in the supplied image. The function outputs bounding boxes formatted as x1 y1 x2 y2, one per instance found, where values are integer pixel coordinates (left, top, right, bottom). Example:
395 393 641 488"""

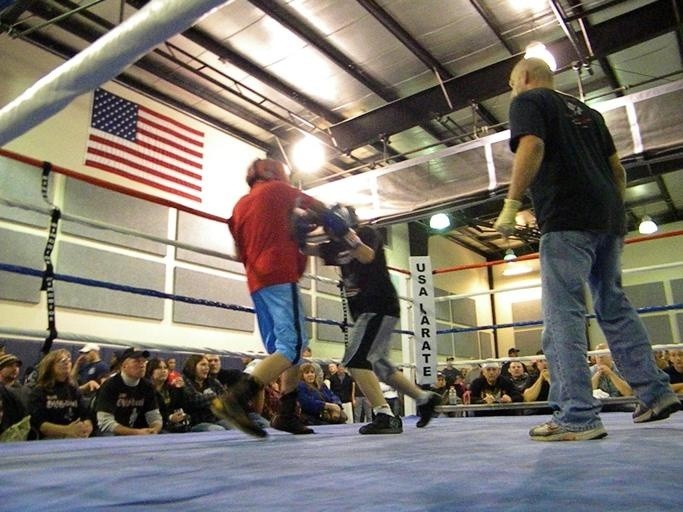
527 421 609 441
359 412 403 434
210 390 269 438
633 393 682 423
444 427 444 432
270 415 312 434
416 391 442 428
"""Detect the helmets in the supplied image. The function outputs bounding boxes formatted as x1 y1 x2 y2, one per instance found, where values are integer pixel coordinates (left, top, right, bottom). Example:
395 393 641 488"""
324 203 361 237
247 157 293 188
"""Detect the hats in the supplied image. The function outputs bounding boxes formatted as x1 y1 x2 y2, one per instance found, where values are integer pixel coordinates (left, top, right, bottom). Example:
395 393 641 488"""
482 358 500 368
78 341 100 354
447 356 454 361
507 348 520 354
0 352 24 370
122 346 151 361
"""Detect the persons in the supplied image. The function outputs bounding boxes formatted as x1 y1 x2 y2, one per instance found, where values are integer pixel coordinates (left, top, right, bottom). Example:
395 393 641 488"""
201 350 252 427
441 355 461 389
503 350 528 376
324 364 358 425
70 342 110 389
297 364 349 424
522 351 551 401
93 347 162 436
584 344 633 400
505 362 531 395
287 204 443 435
142 356 197 433
181 354 235 429
28 351 97 439
0 351 30 440
496 58 679 441
211 157 359 438
165 358 182 387
328 363 338 378
470 357 525 415
656 343 683 389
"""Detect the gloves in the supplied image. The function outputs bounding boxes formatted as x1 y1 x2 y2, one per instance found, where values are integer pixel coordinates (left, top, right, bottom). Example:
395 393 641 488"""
493 196 521 236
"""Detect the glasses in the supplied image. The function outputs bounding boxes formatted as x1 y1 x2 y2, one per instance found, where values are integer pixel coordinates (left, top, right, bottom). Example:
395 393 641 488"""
124 359 148 367
55 358 73 364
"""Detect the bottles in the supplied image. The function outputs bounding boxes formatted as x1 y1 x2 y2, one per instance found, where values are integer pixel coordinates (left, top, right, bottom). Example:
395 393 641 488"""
449 386 457 405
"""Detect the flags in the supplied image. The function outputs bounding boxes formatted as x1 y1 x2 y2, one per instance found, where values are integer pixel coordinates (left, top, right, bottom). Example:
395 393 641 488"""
82 87 206 204
